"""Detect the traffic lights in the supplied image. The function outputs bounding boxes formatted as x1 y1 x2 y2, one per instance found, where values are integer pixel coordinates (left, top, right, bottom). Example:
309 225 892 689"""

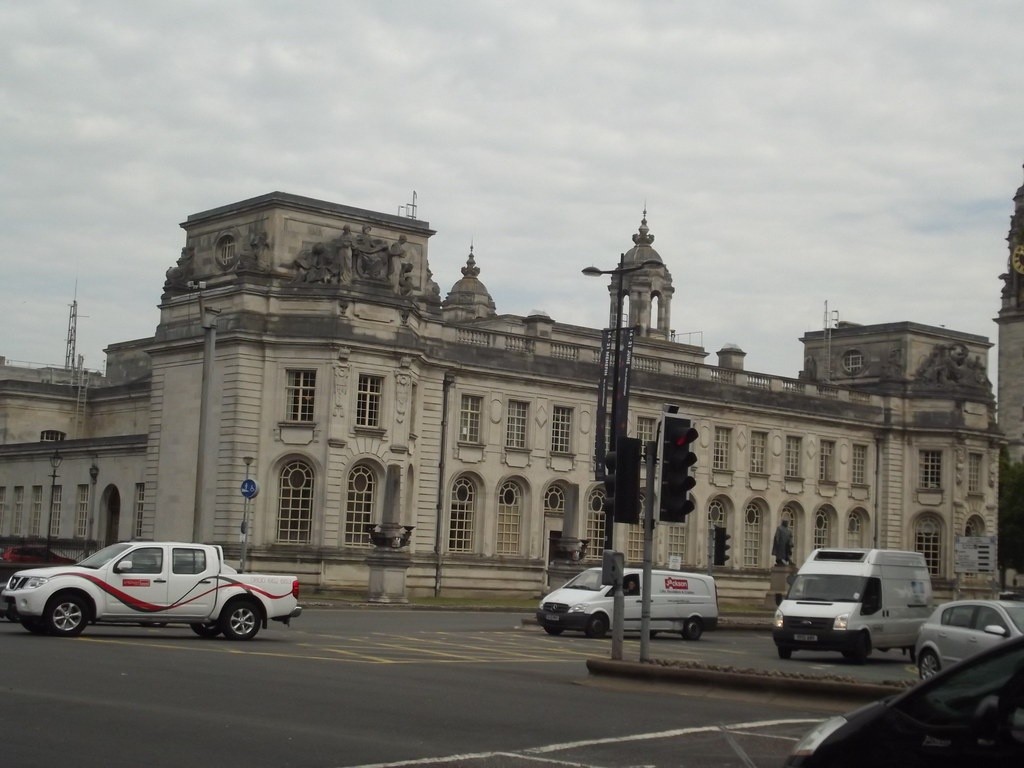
601 434 642 525
656 412 699 527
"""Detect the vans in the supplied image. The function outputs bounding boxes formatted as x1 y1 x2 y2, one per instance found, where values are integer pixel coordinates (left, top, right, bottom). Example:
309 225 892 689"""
536 564 720 639
772 547 936 666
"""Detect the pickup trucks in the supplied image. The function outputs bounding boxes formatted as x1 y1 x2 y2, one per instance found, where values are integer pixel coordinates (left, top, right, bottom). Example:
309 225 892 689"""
0 541 304 642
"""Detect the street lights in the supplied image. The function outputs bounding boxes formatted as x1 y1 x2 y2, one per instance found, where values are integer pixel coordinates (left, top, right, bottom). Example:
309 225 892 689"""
238 453 256 572
45 448 65 559
581 253 667 652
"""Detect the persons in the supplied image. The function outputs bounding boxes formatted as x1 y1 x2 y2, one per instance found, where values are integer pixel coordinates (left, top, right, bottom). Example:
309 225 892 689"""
624 581 638 596
956 456 965 485
916 341 993 388
293 223 415 295
772 519 796 566
989 458 999 488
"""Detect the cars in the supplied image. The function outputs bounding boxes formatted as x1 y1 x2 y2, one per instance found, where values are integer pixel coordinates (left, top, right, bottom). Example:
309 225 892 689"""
0 545 77 566
914 599 1024 678
781 631 1024 767
